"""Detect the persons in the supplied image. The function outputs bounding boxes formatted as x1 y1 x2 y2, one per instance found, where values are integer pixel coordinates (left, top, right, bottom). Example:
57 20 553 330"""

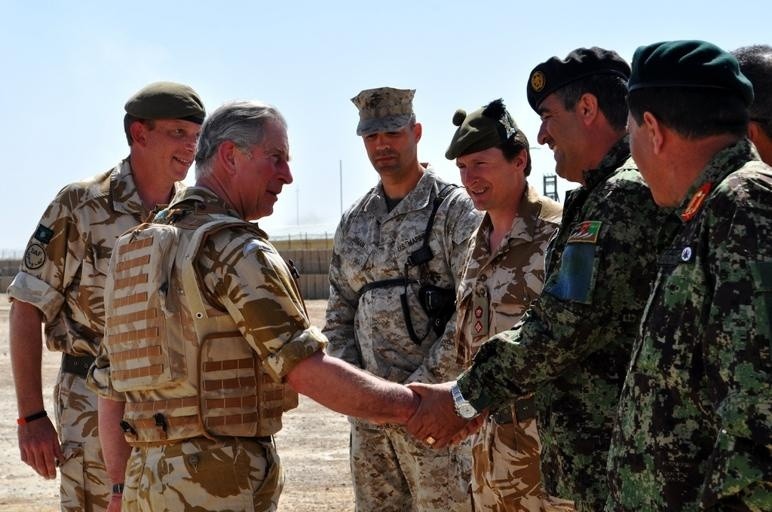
83 101 486 512
320 87 483 512
409 47 673 512
6 80 205 510
405 98 566 511
604 41 769 512
722 45 769 163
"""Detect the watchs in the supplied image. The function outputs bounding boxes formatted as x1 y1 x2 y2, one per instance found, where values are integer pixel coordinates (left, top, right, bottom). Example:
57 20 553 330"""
449 383 481 420
111 483 126 493
14 406 45 426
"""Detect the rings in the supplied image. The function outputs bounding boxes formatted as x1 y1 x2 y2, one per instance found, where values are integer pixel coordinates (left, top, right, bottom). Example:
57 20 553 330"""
425 436 437 445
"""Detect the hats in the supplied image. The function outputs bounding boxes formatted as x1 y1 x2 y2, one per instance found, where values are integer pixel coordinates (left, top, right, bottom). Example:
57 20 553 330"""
525 47 631 110
350 85 417 135
628 38 755 122
444 97 518 161
123 81 207 126
